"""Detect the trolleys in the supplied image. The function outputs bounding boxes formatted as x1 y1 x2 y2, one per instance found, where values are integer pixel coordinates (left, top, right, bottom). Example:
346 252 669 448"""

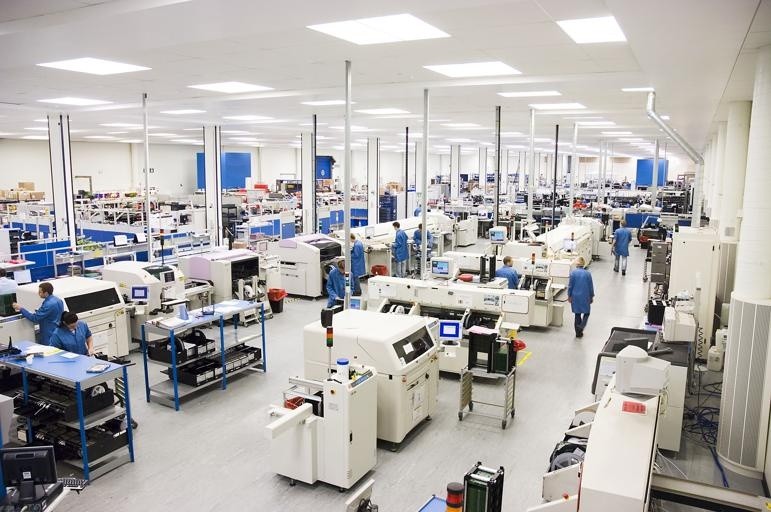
409 249 432 279
642 247 651 283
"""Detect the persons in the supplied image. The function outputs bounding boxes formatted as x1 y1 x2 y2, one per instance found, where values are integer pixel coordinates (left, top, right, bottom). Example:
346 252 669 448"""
0 268 18 294
565 259 595 339
494 256 519 289
609 219 633 276
12 281 64 344
319 221 434 307
47 310 98 357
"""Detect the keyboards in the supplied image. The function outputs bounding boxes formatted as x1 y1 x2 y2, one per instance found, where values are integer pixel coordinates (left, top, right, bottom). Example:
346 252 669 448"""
57 478 89 491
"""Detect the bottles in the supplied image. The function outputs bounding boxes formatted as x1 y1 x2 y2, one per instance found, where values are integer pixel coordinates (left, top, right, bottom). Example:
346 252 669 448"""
708 324 729 370
26 354 32 366
337 355 366 382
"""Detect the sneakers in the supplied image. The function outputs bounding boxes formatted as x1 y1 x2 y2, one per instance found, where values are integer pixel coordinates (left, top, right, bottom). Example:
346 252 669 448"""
352 289 361 296
575 325 584 337
621 269 625 275
613 267 619 272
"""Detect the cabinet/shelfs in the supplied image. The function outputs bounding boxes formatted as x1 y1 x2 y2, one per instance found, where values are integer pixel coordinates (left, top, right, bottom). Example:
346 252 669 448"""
0 336 138 486
140 297 267 413
222 203 238 232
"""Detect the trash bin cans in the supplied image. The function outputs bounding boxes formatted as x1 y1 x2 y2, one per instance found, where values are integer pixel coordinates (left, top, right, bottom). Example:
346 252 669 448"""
640 235 649 249
268 288 287 313
68 265 82 277
371 265 386 275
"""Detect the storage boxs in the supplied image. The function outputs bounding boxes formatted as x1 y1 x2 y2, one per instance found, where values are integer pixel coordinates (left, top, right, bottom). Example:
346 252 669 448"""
0 180 47 203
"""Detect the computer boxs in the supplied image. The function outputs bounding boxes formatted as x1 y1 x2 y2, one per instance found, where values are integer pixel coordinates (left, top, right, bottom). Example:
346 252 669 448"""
10 482 63 512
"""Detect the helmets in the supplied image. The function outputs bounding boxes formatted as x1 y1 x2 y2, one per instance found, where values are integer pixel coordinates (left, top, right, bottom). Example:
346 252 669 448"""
337 256 346 274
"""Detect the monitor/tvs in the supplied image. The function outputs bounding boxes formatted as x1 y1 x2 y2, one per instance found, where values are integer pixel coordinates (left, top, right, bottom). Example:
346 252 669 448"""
478 210 487 220
349 296 367 311
616 346 671 396
180 215 187 225
431 257 454 279
0 445 57 504
439 320 463 345
662 307 696 342
131 285 150 305
489 226 507 243
